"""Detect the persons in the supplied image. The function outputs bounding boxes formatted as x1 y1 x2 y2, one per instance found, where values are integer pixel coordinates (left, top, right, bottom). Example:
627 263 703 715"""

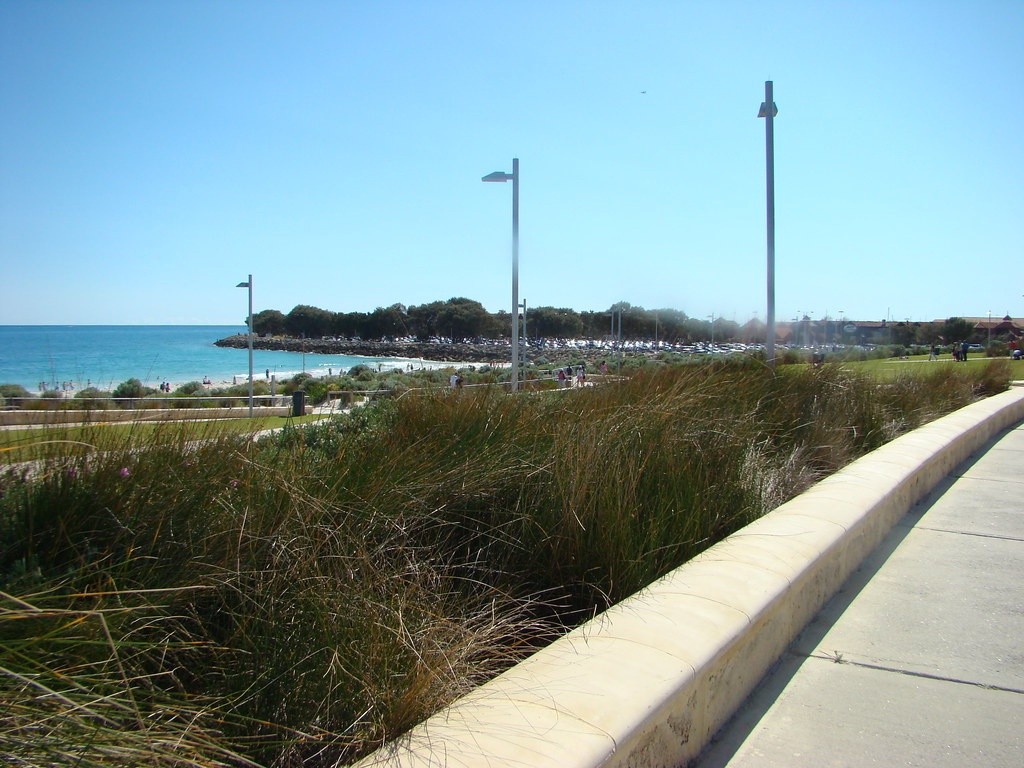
818 345 825 365
450 371 463 389
161 382 170 393
407 363 414 371
928 340 968 361
328 368 346 377
1009 339 1021 360
266 369 269 378
38 379 92 391
600 360 608 375
558 365 585 388
490 359 504 368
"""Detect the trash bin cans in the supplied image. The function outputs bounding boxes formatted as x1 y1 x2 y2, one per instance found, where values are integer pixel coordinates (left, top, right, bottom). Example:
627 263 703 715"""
292 390 306 417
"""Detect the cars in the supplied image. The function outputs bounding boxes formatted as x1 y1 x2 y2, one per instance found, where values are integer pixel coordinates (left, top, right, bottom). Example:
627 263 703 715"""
256 329 986 355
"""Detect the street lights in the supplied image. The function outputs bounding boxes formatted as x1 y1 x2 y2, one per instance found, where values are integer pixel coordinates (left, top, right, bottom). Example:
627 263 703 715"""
654 312 658 351
791 316 799 345
797 310 814 321
705 312 715 354
607 303 614 362
985 310 992 349
236 274 253 418
518 298 527 392
481 158 519 396
756 81 778 368
888 307 889 322
617 308 621 374
838 310 845 319
